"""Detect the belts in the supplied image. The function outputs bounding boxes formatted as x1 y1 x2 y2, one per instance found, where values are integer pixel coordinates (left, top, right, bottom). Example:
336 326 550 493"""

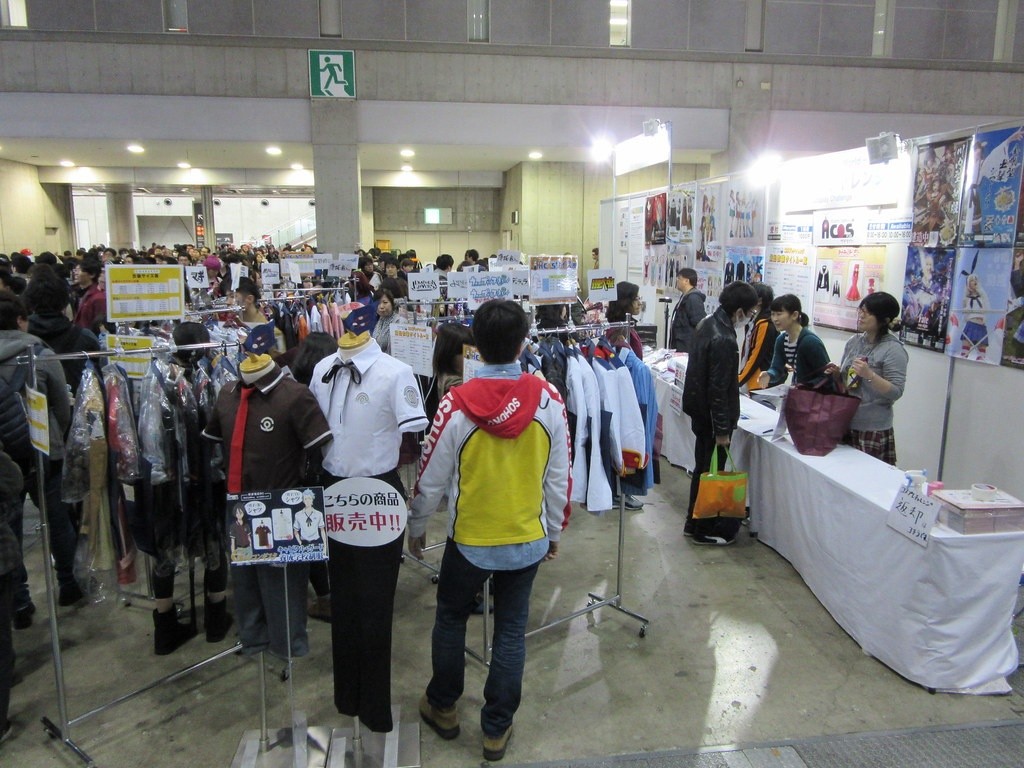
317 468 397 482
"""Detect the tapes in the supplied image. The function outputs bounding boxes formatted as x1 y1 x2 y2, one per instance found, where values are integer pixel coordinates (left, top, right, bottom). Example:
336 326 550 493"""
971 484 997 502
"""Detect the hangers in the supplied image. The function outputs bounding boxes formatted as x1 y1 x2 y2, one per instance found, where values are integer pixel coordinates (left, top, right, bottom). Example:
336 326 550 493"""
264 291 340 324
76 342 232 402
189 309 253 337
525 324 638 374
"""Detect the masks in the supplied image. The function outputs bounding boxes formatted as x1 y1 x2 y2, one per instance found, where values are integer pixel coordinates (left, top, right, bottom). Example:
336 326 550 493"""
732 308 749 330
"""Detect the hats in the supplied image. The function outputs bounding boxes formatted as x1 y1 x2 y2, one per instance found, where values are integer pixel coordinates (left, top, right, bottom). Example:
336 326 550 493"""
202 255 221 271
19 248 71 316
235 277 262 300
172 322 211 364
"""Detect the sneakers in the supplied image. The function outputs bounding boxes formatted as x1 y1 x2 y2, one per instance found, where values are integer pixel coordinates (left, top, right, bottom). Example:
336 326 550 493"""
683 523 693 536
693 526 736 545
58 575 99 607
610 494 644 510
11 601 36 630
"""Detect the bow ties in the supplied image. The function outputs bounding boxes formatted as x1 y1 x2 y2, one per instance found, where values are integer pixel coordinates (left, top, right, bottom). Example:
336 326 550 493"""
728 263 733 266
321 359 363 385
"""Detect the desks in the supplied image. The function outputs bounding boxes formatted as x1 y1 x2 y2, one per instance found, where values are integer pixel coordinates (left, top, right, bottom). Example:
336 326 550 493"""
644 350 1024 693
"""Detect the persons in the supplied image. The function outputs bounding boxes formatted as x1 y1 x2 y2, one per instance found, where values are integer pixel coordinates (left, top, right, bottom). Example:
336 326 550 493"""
729 190 757 238
825 291 909 466
0 442 37 745
666 268 707 353
407 299 574 762
0 289 97 629
371 290 408 356
605 281 644 510
756 294 839 397
220 276 287 359
591 248 599 269
960 273 991 360
682 280 758 545
738 281 789 398
133 322 233 655
308 330 429 733
201 353 334 658
0 242 497 536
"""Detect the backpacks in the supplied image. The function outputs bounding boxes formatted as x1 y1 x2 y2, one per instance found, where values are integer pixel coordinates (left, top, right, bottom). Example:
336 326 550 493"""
0 344 46 461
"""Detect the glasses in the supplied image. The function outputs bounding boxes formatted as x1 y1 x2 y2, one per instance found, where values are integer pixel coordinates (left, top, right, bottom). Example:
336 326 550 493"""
633 297 641 304
856 306 874 318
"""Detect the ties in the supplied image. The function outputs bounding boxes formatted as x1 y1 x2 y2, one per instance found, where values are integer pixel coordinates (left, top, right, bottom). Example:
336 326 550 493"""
227 389 252 495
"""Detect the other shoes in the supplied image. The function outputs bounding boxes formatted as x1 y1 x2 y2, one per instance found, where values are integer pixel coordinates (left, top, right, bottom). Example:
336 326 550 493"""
310 600 333 623
418 690 459 741
481 717 513 761
472 593 495 615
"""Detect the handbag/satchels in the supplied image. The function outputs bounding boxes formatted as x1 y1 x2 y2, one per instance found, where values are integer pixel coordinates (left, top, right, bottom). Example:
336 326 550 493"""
783 355 869 457
690 441 747 520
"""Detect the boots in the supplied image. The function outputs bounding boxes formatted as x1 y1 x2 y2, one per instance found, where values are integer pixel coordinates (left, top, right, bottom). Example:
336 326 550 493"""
206 596 233 643
152 600 198 655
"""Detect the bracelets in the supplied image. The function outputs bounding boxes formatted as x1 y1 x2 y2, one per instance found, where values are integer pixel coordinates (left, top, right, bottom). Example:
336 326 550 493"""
867 372 875 383
